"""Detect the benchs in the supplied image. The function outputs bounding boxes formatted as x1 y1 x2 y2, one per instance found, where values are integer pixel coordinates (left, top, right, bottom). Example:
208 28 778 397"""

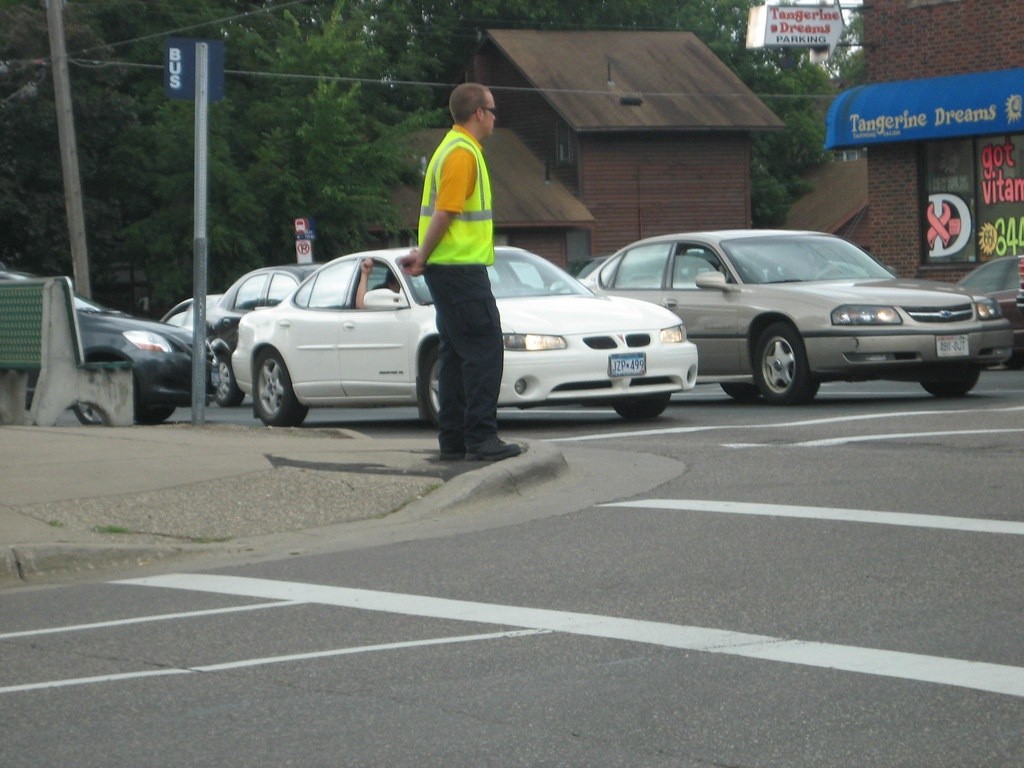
0 275 135 427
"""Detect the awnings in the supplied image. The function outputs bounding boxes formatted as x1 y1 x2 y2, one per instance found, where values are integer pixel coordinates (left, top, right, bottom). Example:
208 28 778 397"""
823 67 1024 151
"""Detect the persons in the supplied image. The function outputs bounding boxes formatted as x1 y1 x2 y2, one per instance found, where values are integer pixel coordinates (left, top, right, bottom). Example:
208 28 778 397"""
398 83 521 463
355 259 399 309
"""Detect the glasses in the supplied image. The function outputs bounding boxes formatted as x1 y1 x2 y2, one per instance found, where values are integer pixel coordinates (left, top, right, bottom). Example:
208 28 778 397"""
473 106 498 117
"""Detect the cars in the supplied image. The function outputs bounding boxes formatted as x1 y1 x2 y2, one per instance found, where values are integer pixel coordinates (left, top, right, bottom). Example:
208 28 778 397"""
1 265 220 424
156 247 703 423
575 229 1019 402
956 255 1024 367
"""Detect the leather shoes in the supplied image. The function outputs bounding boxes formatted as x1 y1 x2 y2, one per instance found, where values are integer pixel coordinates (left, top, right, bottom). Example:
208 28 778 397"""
465 437 521 462
440 440 508 460
